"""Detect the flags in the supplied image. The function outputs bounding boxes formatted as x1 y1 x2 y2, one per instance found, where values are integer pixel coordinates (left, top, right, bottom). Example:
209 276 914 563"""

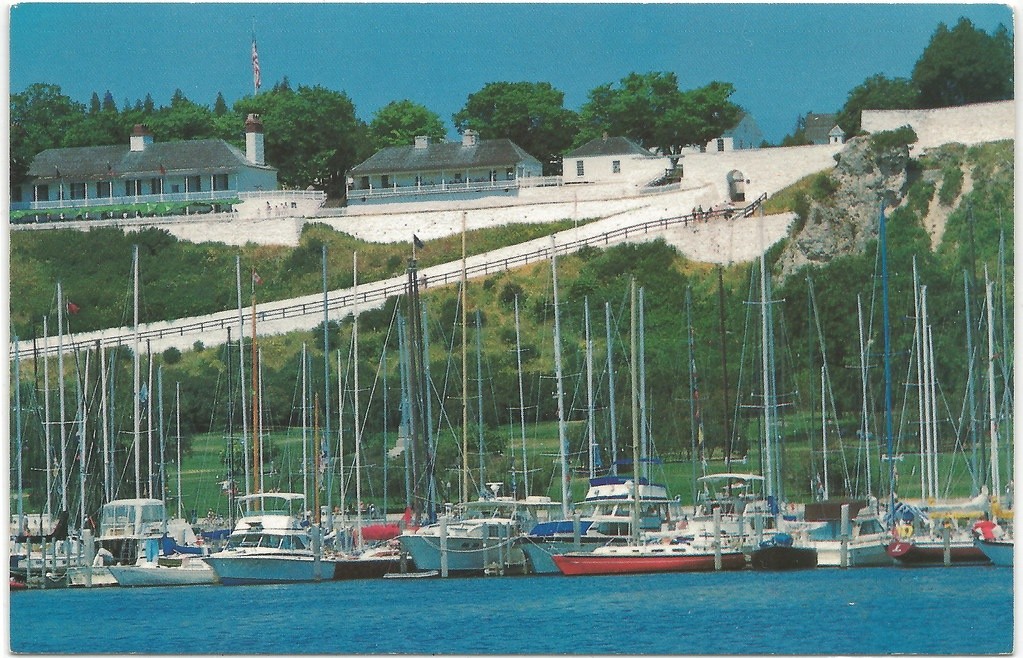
67 301 79 313
252 38 261 87
690 336 704 444
254 272 263 285
413 235 424 248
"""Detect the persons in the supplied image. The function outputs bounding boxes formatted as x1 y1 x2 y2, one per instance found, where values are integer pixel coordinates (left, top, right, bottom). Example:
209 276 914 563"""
370 504 375 512
698 205 703 219
360 501 364 509
207 508 213 518
23 512 30 533
709 200 735 220
423 274 427 288
692 207 696 218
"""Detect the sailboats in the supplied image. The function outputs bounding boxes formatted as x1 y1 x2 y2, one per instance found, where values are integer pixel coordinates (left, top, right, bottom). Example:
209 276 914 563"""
10 196 1013 590
